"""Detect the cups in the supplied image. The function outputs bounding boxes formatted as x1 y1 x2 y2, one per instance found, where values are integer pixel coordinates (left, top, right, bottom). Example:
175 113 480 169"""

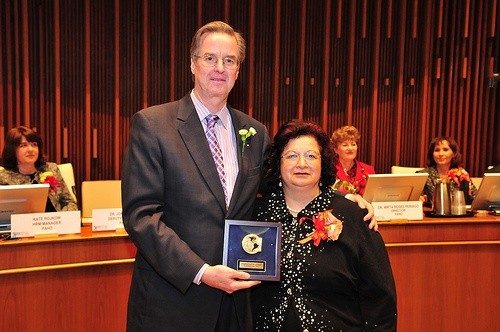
451 190 466 215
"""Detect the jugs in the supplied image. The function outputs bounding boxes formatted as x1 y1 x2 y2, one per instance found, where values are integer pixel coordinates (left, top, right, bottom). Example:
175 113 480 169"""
430 182 450 215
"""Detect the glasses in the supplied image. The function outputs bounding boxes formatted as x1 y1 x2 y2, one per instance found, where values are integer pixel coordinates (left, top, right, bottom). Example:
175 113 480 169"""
194 52 240 70
280 150 322 162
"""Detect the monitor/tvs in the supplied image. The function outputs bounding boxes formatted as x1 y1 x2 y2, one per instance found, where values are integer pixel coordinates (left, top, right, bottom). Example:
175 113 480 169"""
363 172 429 204
0 184 50 230
470 173 500 210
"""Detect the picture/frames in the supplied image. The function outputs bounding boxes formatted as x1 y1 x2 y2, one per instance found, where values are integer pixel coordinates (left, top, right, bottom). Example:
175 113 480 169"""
223 219 282 282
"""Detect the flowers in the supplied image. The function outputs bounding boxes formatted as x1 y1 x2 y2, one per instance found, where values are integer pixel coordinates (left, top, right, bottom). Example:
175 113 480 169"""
37 171 64 192
296 209 343 248
238 126 255 153
448 167 470 188
332 179 359 199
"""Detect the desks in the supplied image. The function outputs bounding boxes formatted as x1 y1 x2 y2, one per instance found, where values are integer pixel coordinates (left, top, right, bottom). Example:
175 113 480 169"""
0 207 500 331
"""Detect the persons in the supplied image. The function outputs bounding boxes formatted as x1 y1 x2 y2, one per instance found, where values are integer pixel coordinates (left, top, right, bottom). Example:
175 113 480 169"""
253 119 398 332
121 21 379 332
330 125 375 197
415 136 478 205
0 126 78 212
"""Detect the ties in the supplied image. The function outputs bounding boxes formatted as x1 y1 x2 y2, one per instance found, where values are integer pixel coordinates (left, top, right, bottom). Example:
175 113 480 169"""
205 114 229 212
